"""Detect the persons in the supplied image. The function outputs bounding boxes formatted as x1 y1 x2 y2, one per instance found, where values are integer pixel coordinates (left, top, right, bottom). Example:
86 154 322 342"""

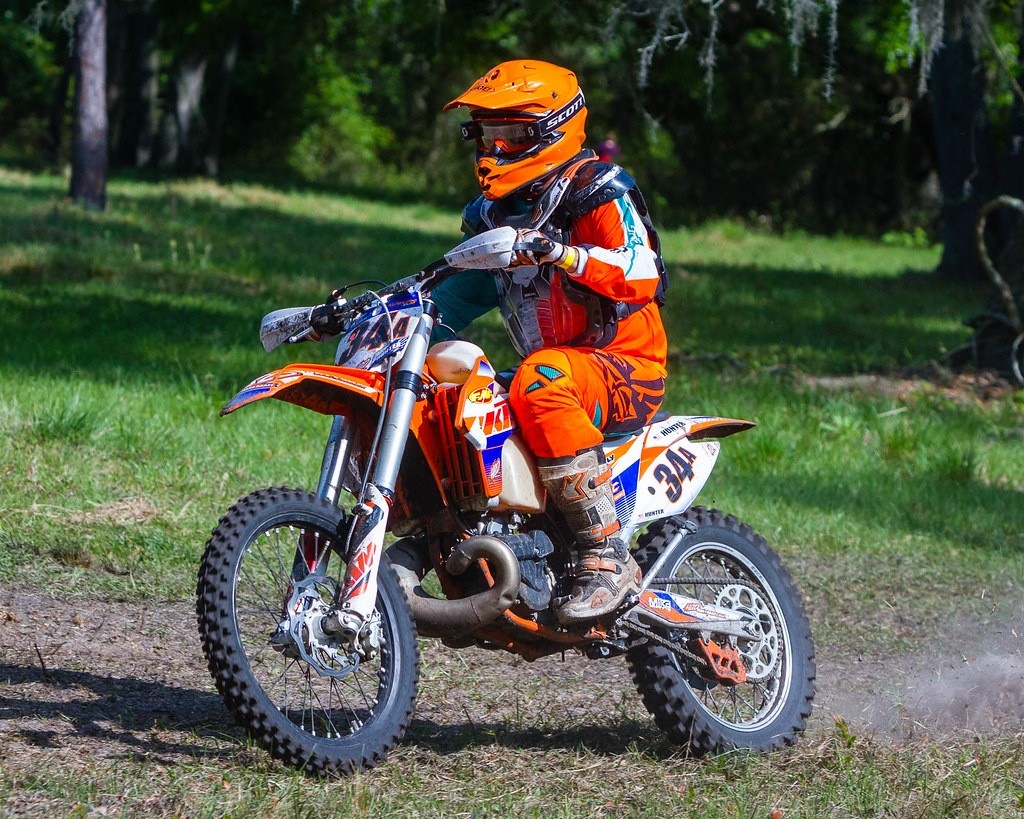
284 59 669 623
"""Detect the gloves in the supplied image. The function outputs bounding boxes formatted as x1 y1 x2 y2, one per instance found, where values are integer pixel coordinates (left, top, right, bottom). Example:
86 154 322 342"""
502 228 565 271
300 307 343 341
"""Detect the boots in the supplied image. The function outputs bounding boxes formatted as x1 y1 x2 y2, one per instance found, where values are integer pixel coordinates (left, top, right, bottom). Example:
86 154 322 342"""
536 445 644 621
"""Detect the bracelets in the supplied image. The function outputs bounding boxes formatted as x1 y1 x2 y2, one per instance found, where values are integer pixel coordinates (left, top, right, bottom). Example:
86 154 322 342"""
558 246 575 269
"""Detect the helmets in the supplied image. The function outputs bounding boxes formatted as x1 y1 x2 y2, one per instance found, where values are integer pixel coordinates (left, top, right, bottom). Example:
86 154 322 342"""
443 59 587 200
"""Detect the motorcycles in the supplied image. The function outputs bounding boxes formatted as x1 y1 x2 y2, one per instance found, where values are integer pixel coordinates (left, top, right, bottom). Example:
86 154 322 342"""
197 225 816 775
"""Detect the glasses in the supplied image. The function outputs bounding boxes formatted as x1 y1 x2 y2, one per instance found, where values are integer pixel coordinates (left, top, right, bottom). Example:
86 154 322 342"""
477 120 538 153
